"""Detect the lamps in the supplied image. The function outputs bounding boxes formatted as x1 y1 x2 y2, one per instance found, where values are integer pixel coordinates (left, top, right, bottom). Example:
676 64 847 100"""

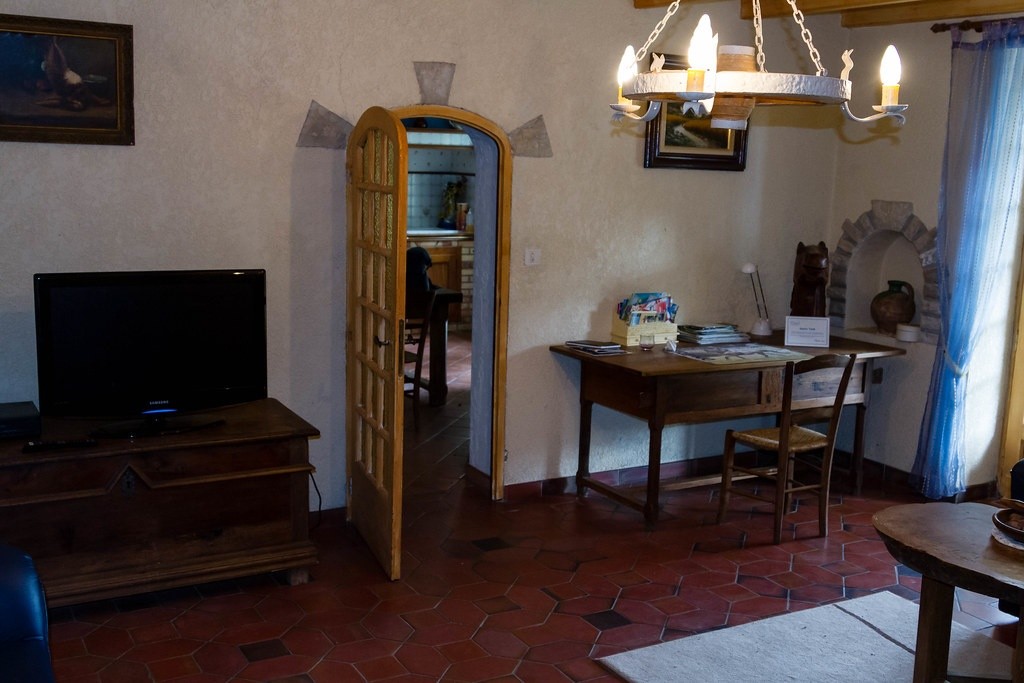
609 0 909 125
742 262 773 335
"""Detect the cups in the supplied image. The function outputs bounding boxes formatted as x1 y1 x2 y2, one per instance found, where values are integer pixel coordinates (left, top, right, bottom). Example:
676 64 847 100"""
639 334 655 351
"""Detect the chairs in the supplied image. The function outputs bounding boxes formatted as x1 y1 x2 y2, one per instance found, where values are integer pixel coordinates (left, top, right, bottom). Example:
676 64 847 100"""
404 290 438 434
719 352 857 545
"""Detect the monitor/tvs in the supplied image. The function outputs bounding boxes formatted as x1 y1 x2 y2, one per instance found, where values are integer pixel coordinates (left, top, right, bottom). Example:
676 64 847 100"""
33 269 268 437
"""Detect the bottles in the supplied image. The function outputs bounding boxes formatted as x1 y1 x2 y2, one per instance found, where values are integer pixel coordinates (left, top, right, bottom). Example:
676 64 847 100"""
870 280 916 332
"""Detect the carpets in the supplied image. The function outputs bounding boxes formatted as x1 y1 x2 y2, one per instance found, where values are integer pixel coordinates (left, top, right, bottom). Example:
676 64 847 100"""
593 590 1016 683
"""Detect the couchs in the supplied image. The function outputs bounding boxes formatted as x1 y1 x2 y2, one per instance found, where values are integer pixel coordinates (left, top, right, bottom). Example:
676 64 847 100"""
0 545 54 683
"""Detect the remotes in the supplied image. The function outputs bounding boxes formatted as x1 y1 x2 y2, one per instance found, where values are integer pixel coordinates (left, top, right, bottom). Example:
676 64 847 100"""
21 439 98 454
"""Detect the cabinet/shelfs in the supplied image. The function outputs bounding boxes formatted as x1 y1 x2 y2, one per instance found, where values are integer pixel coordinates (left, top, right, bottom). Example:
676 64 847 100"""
0 398 320 610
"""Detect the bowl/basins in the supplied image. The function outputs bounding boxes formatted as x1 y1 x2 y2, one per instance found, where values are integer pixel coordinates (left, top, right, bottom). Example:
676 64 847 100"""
992 508 1024 542
896 323 920 342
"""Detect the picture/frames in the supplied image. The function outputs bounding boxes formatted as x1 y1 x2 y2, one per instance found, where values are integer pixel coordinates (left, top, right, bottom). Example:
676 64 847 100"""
644 53 750 172
0 13 136 146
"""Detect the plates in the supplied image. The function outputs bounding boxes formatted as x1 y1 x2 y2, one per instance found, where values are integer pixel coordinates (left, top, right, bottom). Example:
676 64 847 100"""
991 526 1024 550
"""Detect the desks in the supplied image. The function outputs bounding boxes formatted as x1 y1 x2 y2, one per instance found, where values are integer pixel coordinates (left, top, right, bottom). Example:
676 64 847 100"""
873 503 1024 683
549 329 907 531
405 284 463 409
